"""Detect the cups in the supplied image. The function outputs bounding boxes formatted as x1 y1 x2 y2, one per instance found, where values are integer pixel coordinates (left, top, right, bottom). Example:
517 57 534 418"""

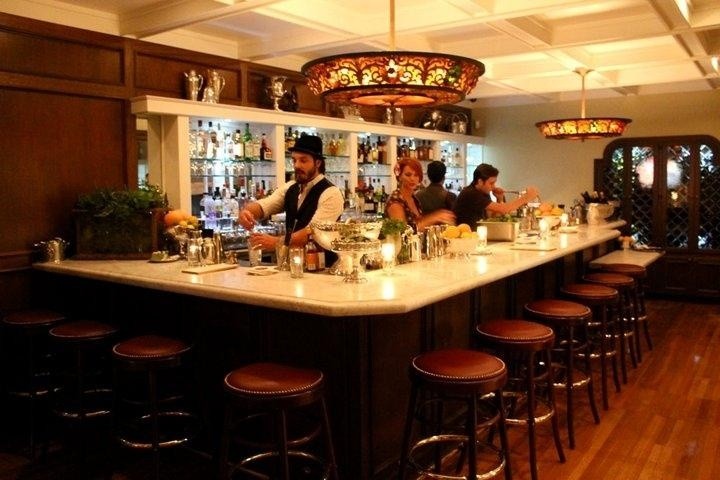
382 107 393 124
557 200 565 209
187 239 200 266
380 243 396 273
41 239 65 263
394 107 403 125
274 240 289 267
247 238 262 266
289 248 304 278
476 226 489 249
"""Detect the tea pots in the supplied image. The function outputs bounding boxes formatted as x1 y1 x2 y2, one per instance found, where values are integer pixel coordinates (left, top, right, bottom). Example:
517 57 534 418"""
445 113 459 133
203 67 227 102
182 69 205 101
454 112 469 134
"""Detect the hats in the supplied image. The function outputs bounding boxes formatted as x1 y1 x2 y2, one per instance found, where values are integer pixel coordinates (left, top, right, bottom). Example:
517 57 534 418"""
288 135 326 158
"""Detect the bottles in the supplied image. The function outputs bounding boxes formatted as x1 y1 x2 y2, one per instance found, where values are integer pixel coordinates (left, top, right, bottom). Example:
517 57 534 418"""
407 234 424 262
364 175 388 217
188 119 271 160
631 222 651 245
569 207 572 217
426 224 447 258
593 190 607 203
574 204 582 219
201 238 215 264
326 173 346 197
284 125 346 157
199 177 267 234
440 148 462 191
356 136 434 162
305 234 317 272
317 244 326 271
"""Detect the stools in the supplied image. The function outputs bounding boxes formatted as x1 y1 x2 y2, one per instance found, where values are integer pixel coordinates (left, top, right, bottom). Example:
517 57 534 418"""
406 349 514 477
222 359 338 478
50 317 113 462
113 334 204 472
474 316 565 475
583 275 641 371
1 316 50 437
558 284 622 416
520 300 601 450
602 263 653 354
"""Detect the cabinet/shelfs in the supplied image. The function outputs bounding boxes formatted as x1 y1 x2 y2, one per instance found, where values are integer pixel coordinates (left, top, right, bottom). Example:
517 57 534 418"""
144 108 284 232
436 131 467 197
277 112 357 223
392 124 440 186
348 123 399 214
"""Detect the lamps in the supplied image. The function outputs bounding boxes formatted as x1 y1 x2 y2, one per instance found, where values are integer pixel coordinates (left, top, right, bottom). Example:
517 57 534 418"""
301 0 486 110
530 66 636 145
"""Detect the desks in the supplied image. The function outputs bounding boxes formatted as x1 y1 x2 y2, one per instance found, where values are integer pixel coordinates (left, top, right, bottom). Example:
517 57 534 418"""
588 247 665 270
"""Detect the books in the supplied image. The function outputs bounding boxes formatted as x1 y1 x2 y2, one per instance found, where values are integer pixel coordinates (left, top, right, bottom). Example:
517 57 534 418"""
182 262 239 276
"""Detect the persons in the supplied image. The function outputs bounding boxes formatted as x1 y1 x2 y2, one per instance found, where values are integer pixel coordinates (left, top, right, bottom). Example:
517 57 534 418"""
239 132 344 265
382 157 457 241
413 161 459 227
454 162 539 232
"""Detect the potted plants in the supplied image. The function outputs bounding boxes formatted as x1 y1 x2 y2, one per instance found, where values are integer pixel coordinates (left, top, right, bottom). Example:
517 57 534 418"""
67 182 173 262
379 218 405 267
